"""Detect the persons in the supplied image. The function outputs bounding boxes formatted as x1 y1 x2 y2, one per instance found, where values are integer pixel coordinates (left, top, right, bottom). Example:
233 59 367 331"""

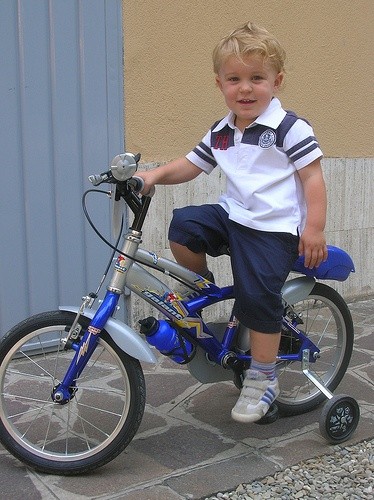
133 20 327 423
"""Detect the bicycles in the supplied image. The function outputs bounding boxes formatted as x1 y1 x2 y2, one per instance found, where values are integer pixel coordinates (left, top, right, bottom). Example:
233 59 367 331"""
0 153 361 475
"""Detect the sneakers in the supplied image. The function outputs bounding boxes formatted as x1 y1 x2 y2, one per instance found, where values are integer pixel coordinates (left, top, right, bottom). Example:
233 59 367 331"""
171 270 214 304
230 370 280 423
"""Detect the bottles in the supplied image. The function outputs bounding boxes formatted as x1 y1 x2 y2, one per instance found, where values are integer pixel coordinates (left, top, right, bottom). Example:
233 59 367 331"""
138 315 197 365
